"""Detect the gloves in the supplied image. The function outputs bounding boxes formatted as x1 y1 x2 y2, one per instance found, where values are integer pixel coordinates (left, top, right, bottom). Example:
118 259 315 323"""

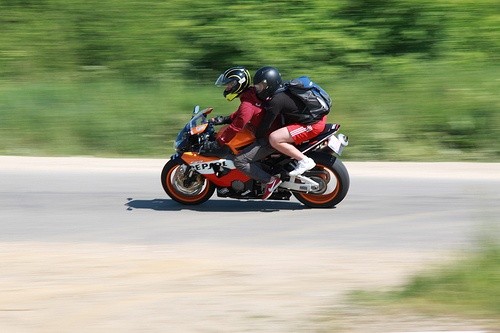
211 140 223 153
213 114 228 127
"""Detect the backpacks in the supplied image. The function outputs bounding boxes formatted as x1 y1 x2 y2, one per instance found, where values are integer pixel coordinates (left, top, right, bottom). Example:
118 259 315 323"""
274 76 332 124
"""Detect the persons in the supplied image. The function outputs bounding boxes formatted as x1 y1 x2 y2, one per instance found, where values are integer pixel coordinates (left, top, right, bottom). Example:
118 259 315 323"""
199 66 332 200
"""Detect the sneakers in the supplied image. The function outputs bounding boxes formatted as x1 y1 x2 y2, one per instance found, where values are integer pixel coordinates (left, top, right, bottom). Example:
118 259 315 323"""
288 158 315 177
262 176 282 200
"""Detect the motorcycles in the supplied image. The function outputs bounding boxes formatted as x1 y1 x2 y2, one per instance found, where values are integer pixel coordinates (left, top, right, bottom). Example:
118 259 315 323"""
160 105 350 208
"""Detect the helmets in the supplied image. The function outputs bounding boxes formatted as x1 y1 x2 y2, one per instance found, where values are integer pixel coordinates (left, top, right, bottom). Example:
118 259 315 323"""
253 67 282 100
214 66 251 101
